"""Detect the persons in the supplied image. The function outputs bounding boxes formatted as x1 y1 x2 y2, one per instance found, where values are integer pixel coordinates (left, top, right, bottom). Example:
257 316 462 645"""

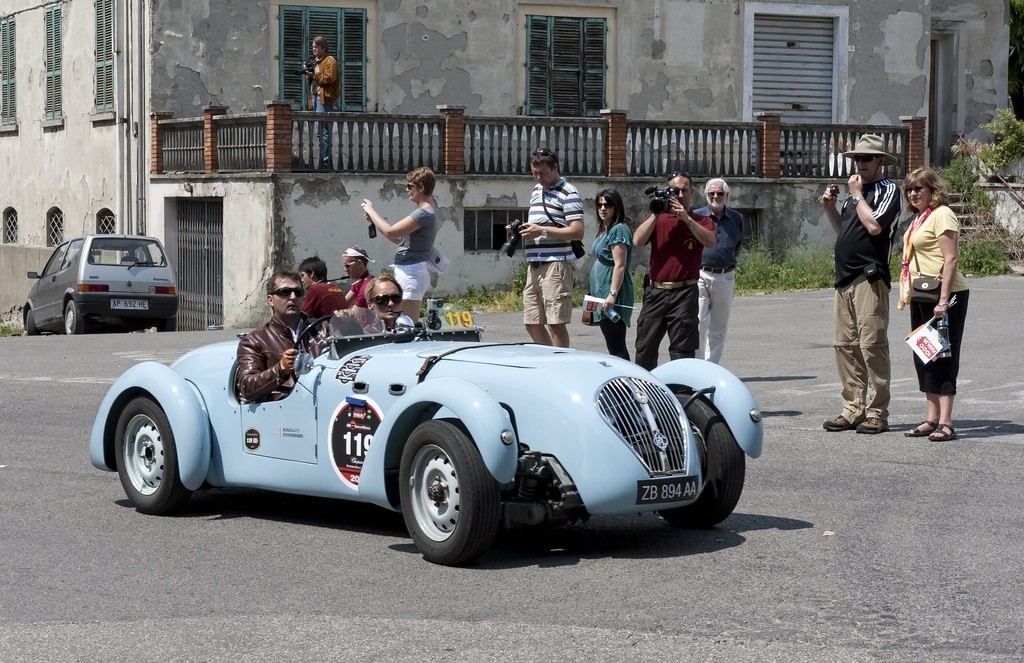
692 177 745 363
903 166 970 442
360 166 440 324
820 134 901 435
504 147 585 348
341 245 376 327
363 275 403 335
236 269 354 403
299 256 349 323
632 170 718 372
304 35 340 168
588 188 634 361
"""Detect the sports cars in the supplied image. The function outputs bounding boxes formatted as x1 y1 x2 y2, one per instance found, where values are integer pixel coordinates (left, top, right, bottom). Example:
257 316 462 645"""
89 310 764 565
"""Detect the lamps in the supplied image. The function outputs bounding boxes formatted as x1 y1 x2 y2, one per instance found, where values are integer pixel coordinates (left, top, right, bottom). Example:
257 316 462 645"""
184 181 192 193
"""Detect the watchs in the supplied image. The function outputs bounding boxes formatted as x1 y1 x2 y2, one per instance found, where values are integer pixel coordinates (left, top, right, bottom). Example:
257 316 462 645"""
851 197 863 206
542 227 548 239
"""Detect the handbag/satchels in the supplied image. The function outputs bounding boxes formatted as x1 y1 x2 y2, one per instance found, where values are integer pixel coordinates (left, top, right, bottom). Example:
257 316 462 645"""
910 277 943 302
581 309 600 326
571 239 586 259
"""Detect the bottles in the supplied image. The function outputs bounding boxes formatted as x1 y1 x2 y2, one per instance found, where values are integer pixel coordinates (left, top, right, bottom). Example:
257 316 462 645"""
937 313 952 358
606 308 621 323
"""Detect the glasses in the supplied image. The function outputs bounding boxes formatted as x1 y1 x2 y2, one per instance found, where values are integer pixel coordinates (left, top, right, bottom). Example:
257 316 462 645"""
596 202 613 209
368 295 404 306
407 183 415 190
905 184 927 194
667 171 692 183
532 149 556 162
707 192 725 197
854 154 885 163
272 288 305 298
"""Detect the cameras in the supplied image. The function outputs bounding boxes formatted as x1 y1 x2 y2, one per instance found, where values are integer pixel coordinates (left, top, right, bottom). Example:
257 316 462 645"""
830 185 839 195
427 299 444 311
501 220 526 258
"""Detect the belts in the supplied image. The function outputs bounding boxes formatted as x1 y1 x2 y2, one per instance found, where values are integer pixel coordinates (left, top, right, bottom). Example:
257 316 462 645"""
700 266 736 274
531 261 548 267
652 278 698 289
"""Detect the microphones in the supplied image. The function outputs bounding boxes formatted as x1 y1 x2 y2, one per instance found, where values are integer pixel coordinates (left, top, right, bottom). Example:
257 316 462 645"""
644 186 657 195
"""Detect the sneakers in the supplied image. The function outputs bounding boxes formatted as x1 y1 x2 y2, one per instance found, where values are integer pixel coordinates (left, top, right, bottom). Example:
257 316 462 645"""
823 413 865 431
856 417 889 434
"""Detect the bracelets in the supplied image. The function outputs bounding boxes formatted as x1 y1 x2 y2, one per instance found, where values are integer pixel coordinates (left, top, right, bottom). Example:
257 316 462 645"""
609 290 617 298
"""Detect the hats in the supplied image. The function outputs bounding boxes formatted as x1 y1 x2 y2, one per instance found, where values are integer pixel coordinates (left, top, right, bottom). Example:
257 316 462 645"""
341 248 376 262
843 134 899 166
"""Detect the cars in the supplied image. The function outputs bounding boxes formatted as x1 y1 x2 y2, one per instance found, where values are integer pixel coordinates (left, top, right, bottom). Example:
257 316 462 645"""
23 235 179 336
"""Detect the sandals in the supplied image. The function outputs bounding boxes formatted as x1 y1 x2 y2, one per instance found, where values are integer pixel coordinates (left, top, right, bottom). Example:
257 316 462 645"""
928 423 956 440
904 420 939 436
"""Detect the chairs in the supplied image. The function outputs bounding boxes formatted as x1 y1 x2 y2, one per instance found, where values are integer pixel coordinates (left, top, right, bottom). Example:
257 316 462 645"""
122 257 139 266
